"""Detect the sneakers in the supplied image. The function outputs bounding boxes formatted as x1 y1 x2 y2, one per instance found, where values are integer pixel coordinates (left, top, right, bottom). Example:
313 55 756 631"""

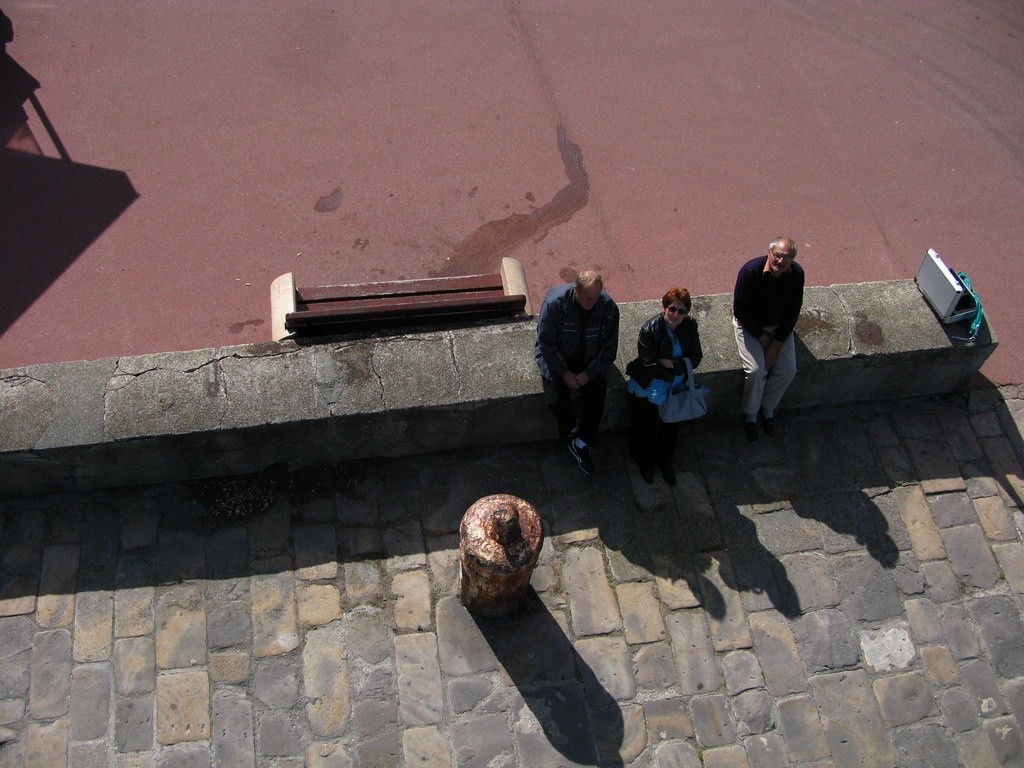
567 439 592 475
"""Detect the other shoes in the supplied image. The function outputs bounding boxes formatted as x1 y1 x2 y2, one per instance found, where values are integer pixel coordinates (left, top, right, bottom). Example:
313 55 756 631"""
656 457 673 485
758 411 774 437
744 418 758 442
638 460 654 485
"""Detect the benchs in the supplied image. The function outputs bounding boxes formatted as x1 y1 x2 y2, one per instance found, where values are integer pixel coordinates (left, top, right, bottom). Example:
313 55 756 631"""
270 256 533 345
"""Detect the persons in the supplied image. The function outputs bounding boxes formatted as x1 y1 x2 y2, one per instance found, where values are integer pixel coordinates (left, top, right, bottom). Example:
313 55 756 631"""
625 288 703 487
732 236 805 444
534 270 620 476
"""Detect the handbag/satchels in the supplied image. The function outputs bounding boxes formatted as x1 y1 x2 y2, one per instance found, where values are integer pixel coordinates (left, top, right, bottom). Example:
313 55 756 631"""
658 358 707 423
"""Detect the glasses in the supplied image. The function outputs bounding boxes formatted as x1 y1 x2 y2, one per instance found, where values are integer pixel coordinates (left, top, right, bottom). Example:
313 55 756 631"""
772 250 793 260
666 306 686 314
